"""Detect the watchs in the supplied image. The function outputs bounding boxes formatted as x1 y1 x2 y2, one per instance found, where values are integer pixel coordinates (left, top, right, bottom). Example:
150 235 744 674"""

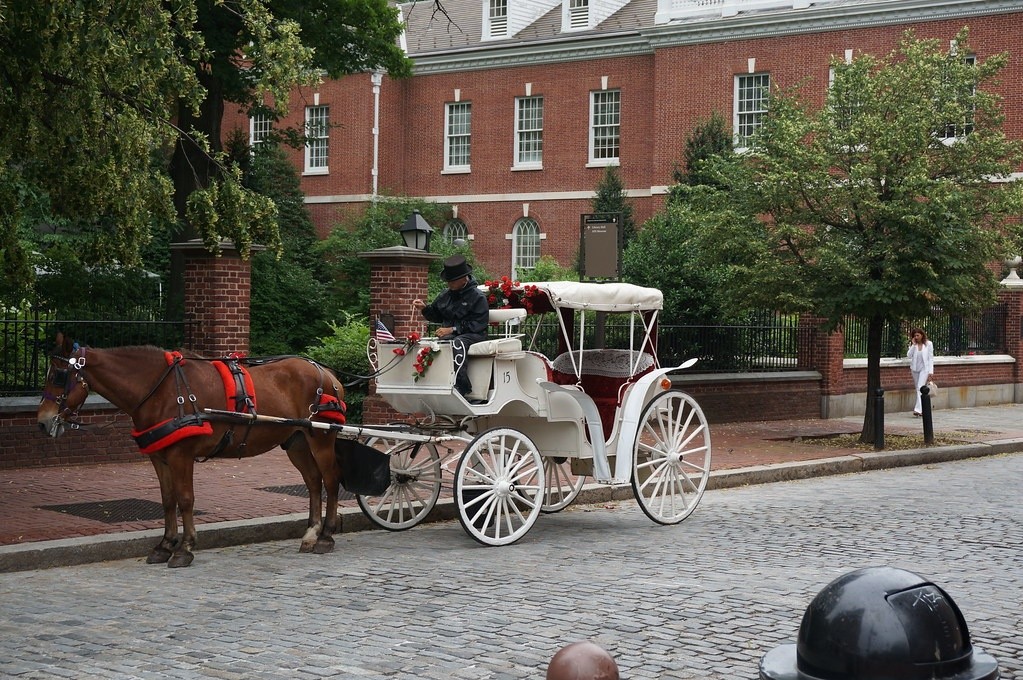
453 326 456 334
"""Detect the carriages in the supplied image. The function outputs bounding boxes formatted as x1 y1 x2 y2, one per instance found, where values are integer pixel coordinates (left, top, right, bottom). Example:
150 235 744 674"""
35 280 713 569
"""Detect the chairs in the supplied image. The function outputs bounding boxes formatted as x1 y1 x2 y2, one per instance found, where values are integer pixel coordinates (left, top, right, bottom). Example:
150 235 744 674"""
467 308 527 355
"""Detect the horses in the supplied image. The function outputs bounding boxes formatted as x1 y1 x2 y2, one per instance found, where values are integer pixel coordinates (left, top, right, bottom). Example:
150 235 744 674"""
37 332 346 568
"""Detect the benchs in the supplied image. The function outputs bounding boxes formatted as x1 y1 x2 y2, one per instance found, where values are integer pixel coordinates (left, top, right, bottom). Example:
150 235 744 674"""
552 348 654 407
516 350 579 397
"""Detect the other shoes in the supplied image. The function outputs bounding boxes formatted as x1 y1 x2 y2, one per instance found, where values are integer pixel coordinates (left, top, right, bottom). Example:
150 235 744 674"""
913 411 923 418
457 389 472 395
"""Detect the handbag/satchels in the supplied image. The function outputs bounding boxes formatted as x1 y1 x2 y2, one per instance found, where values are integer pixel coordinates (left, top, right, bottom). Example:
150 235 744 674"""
926 381 938 395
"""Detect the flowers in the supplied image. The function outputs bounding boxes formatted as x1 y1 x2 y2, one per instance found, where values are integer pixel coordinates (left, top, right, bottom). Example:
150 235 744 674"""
393 332 420 356
412 338 441 382
484 276 542 326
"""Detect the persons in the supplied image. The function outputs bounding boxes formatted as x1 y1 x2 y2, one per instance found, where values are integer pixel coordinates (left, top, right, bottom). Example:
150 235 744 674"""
907 328 933 416
412 255 489 396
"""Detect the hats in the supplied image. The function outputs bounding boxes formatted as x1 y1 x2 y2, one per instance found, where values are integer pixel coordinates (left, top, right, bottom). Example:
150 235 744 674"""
441 255 473 282
